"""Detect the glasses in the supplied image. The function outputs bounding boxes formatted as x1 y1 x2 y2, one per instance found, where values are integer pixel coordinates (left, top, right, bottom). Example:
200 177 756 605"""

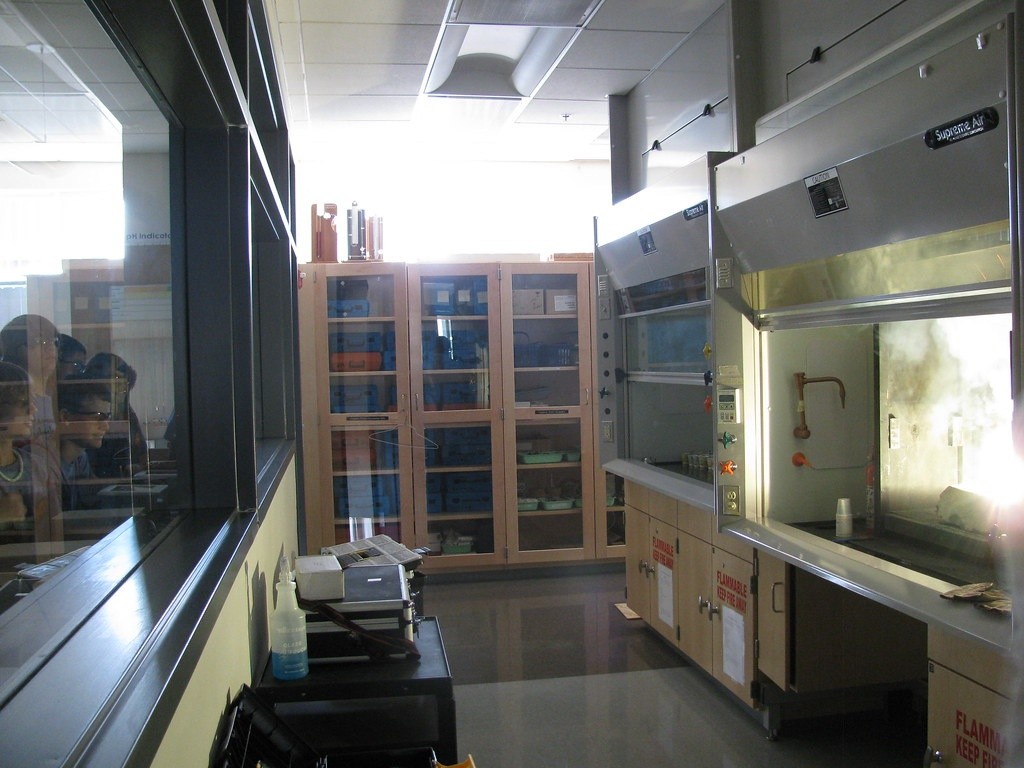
0 395 38 408
77 409 113 422
63 359 87 374
15 334 60 358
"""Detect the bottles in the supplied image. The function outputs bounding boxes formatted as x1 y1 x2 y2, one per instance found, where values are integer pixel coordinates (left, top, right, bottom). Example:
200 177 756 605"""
269 559 309 679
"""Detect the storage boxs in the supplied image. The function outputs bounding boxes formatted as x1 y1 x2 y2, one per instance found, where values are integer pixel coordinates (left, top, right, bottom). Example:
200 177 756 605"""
294 534 428 664
329 278 617 556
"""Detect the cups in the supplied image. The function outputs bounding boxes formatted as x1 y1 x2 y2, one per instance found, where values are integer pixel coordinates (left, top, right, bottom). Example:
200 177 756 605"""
366 216 383 261
682 453 713 470
347 201 366 260
836 498 853 537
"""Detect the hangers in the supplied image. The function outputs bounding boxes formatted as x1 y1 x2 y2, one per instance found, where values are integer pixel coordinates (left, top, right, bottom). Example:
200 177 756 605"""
368 409 440 450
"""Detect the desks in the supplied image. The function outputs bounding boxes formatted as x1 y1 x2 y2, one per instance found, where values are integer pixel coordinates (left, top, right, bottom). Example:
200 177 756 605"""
253 616 458 768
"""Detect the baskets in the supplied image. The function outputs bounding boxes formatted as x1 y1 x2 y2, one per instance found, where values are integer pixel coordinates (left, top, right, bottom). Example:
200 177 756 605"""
514 331 543 367
547 342 571 366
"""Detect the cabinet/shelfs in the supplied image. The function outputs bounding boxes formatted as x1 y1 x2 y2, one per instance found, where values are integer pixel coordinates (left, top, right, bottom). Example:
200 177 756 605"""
677 501 760 708
624 475 678 649
0 269 176 576
923 619 1023 768
311 263 503 573
756 547 921 692
499 261 628 565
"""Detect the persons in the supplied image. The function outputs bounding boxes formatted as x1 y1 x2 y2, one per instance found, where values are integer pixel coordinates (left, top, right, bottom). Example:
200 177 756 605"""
0 315 152 583
